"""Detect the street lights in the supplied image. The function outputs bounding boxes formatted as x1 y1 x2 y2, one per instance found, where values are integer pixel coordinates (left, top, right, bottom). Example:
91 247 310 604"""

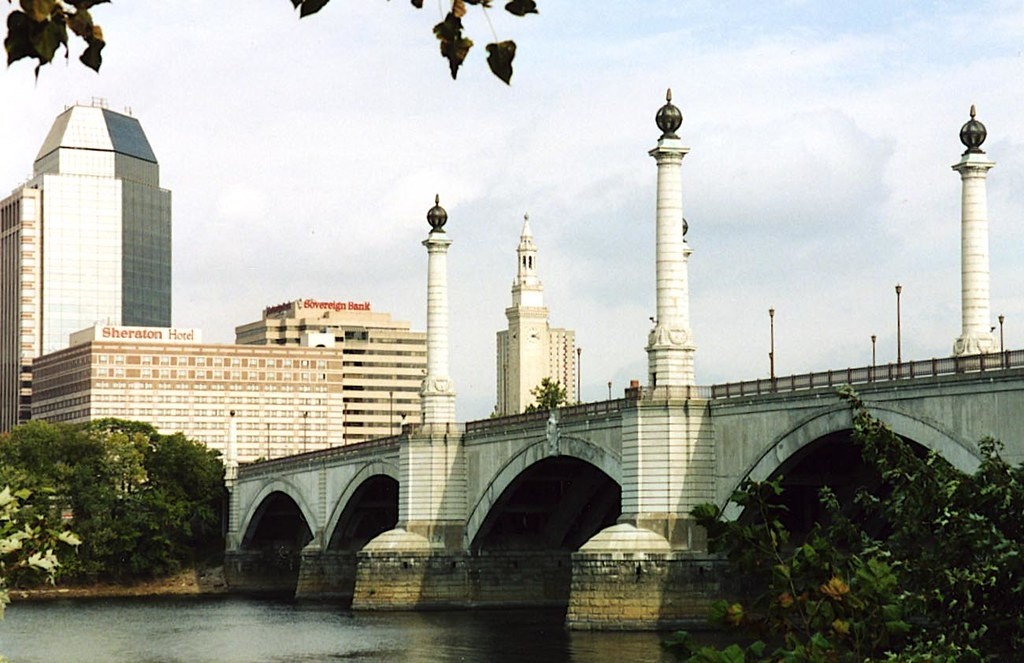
997 313 1006 369
648 84 700 388
767 307 775 392
303 410 309 451
895 283 904 377
576 347 583 405
608 381 613 399
949 101 998 356
871 335 880 380
419 191 461 423
388 390 394 436
